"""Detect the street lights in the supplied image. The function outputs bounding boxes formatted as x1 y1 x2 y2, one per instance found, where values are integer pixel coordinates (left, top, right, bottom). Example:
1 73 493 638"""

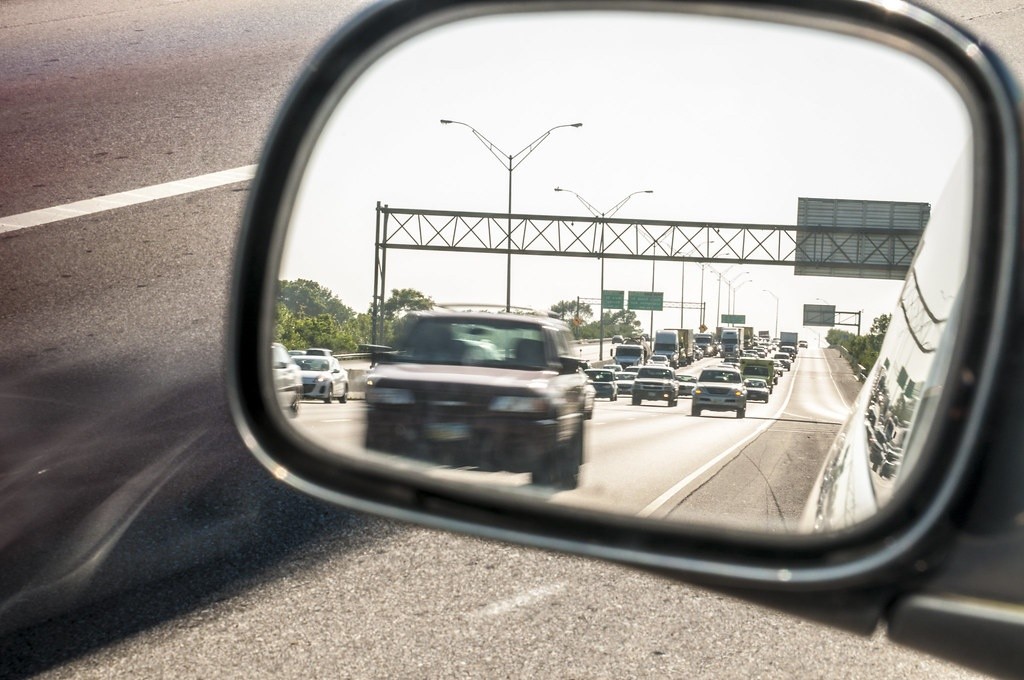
440 118 583 360
679 251 779 338
661 240 714 329
803 326 820 348
816 298 834 329
552 187 654 360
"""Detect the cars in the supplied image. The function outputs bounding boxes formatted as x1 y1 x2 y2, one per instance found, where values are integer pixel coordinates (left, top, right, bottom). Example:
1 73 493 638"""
577 366 596 420
584 326 808 419
269 343 350 418
357 312 590 493
